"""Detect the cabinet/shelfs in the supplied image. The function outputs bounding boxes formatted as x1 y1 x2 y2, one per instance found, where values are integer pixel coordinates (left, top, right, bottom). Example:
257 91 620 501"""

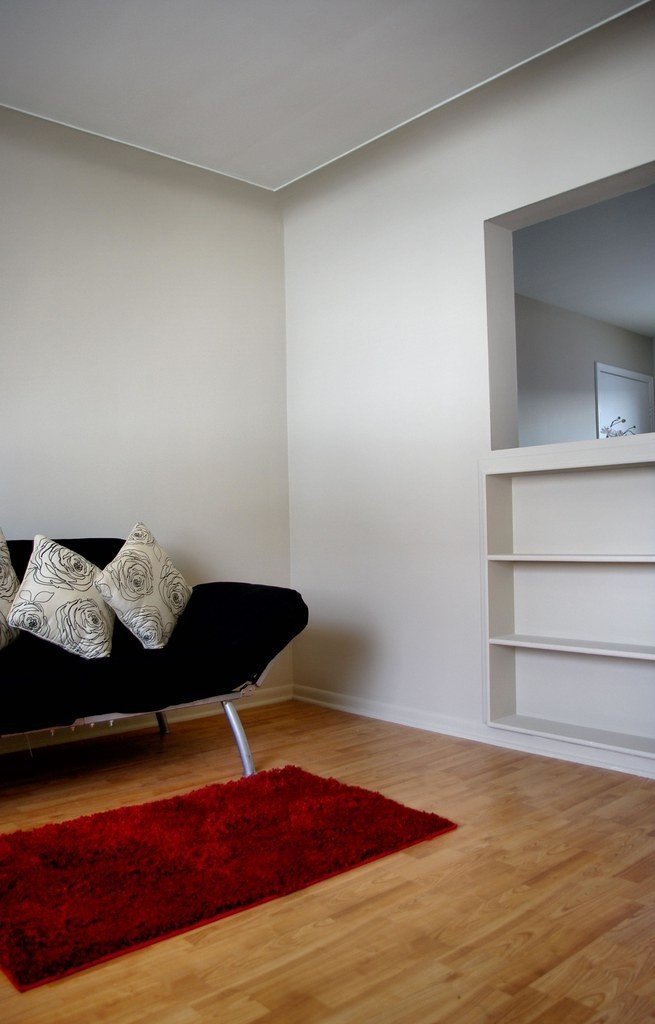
486 433 655 762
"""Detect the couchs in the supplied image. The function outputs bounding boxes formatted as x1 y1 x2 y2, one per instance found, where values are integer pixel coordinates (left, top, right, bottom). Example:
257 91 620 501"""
0 539 309 777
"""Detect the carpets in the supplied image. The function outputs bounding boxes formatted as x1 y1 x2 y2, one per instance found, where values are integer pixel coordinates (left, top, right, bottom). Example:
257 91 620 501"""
0 764 459 994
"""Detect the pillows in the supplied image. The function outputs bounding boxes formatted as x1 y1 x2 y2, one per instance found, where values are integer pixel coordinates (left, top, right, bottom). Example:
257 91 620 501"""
5 533 114 659
0 529 20 651
94 523 192 651
